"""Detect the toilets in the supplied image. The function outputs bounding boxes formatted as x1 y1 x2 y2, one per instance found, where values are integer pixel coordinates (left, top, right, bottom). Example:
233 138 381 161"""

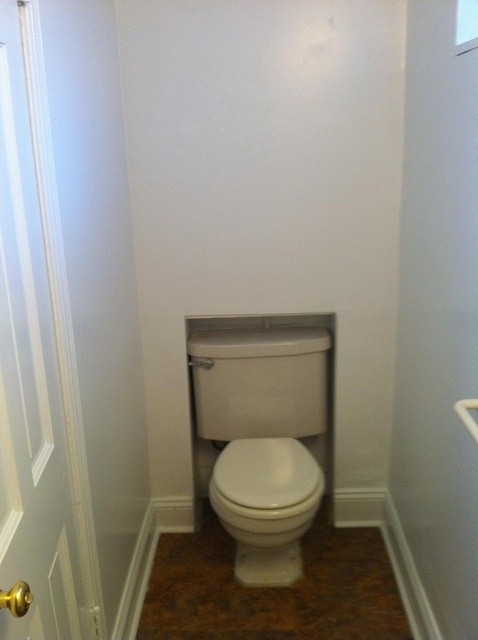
187 326 333 586
